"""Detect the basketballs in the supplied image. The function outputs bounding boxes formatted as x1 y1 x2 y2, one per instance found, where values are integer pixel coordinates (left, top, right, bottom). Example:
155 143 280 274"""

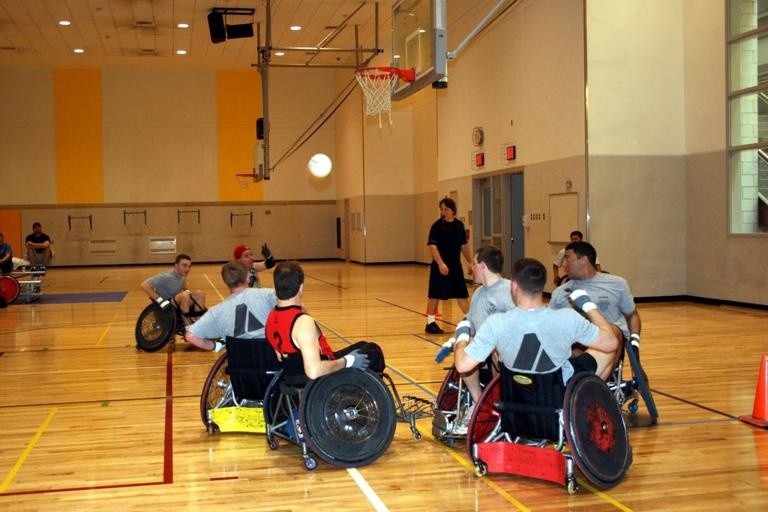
308 154 332 177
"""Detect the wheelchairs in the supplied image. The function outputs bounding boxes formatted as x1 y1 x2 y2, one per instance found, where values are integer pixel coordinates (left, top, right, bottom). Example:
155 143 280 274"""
0 275 21 308
466 362 630 494
607 336 658 425
263 351 434 470
200 336 282 435
135 296 208 352
557 271 612 288
432 354 493 448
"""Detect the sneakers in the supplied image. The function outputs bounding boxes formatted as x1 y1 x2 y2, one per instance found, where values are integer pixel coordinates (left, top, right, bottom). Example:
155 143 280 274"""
39 265 45 276
31 266 39 277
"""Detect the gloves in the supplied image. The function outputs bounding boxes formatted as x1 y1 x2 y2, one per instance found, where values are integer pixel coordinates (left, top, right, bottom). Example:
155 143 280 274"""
213 341 227 353
455 320 471 340
347 347 370 372
631 337 641 362
569 289 593 312
261 243 271 260
435 341 454 363
158 298 176 312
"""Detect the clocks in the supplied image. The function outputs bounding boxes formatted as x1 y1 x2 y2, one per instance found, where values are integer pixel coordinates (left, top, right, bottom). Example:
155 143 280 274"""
471 126 485 145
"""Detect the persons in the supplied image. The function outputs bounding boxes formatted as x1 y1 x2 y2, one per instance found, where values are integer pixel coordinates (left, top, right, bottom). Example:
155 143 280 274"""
425 197 474 335
265 259 386 380
0 232 13 276
185 260 277 354
232 243 276 289
454 257 624 401
25 222 56 272
435 246 514 435
140 254 206 327
549 240 641 365
553 229 608 286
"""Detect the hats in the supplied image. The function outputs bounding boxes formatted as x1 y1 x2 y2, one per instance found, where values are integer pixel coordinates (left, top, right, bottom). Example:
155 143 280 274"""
234 245 251 259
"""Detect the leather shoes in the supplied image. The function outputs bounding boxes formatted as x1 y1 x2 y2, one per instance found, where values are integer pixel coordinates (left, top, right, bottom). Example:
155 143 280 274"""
426 322 445 335
